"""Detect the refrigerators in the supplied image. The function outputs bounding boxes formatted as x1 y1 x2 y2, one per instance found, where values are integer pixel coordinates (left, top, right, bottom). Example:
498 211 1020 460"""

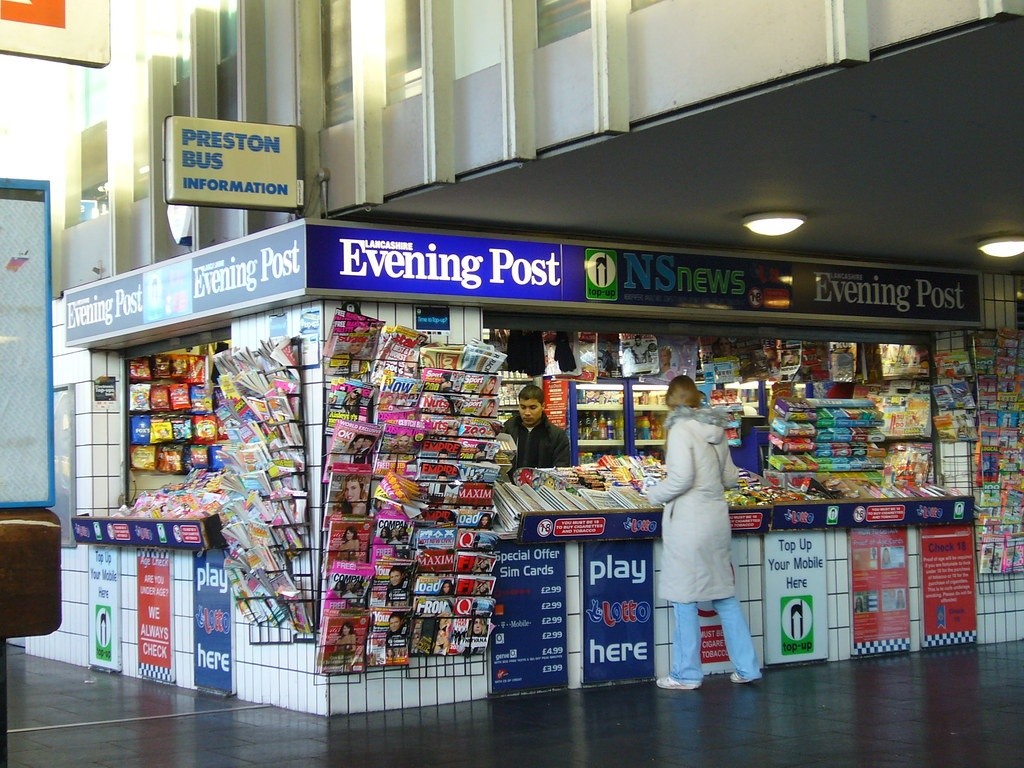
542 374 672 468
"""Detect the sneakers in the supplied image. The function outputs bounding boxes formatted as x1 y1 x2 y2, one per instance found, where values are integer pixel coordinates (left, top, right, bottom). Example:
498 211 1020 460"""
730 672 763 683
656 676 703 689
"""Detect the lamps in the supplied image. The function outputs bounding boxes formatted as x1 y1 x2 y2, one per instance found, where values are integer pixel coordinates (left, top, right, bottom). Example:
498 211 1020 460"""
740 212 808 237
975 235 1024 258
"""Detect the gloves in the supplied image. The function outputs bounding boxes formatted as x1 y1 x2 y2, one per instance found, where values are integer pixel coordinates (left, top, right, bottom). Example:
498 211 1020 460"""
554 333 577 372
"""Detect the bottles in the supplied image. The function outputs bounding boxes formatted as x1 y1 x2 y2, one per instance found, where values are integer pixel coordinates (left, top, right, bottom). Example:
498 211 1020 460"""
578 411 624 440
635 448 665 465
578 447 623 465
634 416 667 441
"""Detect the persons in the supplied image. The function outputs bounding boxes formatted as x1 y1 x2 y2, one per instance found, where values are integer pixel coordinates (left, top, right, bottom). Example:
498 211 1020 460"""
881 548 894 569
631 335 653 364
855 597 863 612
655 347 677 380
501 385 571 471
644 374 764 690
896 590 905 608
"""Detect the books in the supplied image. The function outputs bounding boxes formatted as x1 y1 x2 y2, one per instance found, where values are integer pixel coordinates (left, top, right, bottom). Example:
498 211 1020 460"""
208 310 509 675
